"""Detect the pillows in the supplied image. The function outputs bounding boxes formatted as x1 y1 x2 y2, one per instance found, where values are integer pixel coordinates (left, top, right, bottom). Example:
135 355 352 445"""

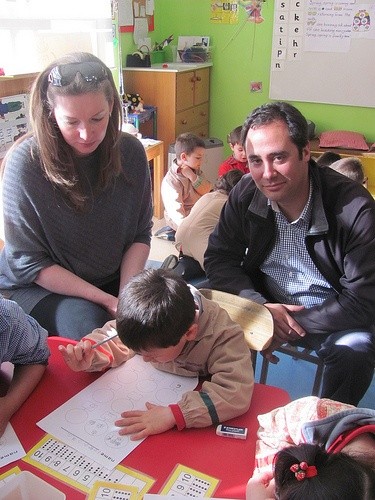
319 131 370 150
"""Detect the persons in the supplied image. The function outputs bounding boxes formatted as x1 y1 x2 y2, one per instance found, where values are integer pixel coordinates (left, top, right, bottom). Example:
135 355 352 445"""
0 294 51 438
315 151 342 168
161 133 210 232
58 269 254 441
193 101 375 406
218 125 250 180
329 157 364 185
174 168 244 272
246 396 375 500
0 52 153 341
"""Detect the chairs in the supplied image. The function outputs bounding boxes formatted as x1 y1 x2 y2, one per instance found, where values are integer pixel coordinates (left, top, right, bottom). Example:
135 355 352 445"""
198 288 275 379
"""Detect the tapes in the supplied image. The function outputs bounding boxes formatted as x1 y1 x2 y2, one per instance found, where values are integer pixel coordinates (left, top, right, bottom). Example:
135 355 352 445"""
132 51 143 60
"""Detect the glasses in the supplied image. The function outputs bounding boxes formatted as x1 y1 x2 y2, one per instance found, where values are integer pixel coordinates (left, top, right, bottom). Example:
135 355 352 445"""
47 62 109 87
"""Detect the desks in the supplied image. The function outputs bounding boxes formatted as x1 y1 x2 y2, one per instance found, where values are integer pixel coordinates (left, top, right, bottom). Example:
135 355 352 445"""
144 140 165 220
0 336 292 500
309 138 375 200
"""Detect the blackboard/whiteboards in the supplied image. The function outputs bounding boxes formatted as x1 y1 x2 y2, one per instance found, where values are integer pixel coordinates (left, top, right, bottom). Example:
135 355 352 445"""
268 0 375 109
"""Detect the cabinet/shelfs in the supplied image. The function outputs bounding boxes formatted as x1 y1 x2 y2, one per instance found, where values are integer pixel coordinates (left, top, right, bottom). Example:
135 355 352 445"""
122 62 213 144
122 105 157 140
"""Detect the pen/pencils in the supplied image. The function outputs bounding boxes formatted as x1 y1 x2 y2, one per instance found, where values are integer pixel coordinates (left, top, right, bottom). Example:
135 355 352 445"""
84 334 118 355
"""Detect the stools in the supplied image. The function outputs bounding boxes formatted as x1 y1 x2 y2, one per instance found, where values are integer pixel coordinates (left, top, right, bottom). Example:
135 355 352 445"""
251 339 326 398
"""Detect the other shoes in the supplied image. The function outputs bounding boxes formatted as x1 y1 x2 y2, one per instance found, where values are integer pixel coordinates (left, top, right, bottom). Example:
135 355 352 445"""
157 255 194 281
154 226 175 241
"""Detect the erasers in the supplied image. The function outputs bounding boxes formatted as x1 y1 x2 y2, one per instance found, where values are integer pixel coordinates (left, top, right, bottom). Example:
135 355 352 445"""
215 424 248 440
